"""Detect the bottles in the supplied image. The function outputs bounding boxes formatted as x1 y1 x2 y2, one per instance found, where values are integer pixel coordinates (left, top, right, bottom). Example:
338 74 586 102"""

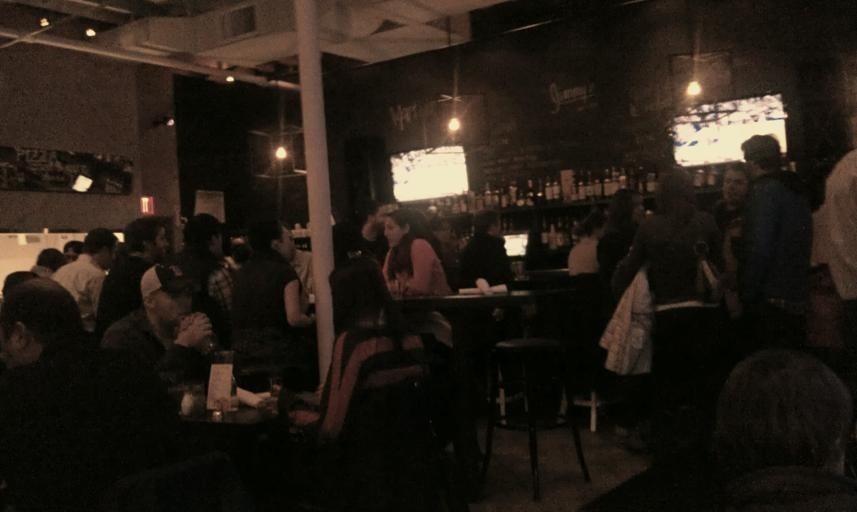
487 219 579 253
692 167 718 188
424 164 654 218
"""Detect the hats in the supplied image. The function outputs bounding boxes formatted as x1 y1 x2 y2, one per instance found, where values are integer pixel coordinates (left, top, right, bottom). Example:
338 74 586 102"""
140 263 207 298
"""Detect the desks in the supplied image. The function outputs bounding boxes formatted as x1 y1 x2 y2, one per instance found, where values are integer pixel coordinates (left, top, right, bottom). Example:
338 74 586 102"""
182 400 271 444
391 291 532 306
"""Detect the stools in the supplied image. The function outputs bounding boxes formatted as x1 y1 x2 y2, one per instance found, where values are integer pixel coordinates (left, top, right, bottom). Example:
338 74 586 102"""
480 338 627 501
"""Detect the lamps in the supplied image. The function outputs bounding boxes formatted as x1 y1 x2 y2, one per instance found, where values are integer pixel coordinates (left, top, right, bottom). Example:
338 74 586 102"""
424 16 489 149
250 75 306 182
664 0 736 123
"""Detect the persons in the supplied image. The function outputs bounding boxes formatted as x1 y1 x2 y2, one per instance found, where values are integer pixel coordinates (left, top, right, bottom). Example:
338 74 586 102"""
1 150 857 512
674 95 788 163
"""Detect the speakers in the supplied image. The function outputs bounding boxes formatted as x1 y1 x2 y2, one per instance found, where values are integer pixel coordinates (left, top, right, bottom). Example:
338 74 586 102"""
801 49 857 154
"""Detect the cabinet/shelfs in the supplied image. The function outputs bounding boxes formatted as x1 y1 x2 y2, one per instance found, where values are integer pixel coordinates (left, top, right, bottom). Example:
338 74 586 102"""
417 167 804 287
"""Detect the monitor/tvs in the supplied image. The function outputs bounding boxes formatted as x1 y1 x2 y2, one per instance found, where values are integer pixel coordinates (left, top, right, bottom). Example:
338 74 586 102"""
389 145 470 203
665 89 792 167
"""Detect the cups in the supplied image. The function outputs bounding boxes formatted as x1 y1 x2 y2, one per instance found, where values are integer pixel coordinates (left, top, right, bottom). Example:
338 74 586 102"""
181 392 205 417
511 260 524 279
181 321 219 353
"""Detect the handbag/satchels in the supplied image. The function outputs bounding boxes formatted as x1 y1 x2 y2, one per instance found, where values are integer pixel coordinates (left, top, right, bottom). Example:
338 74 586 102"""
695 255 726 304
